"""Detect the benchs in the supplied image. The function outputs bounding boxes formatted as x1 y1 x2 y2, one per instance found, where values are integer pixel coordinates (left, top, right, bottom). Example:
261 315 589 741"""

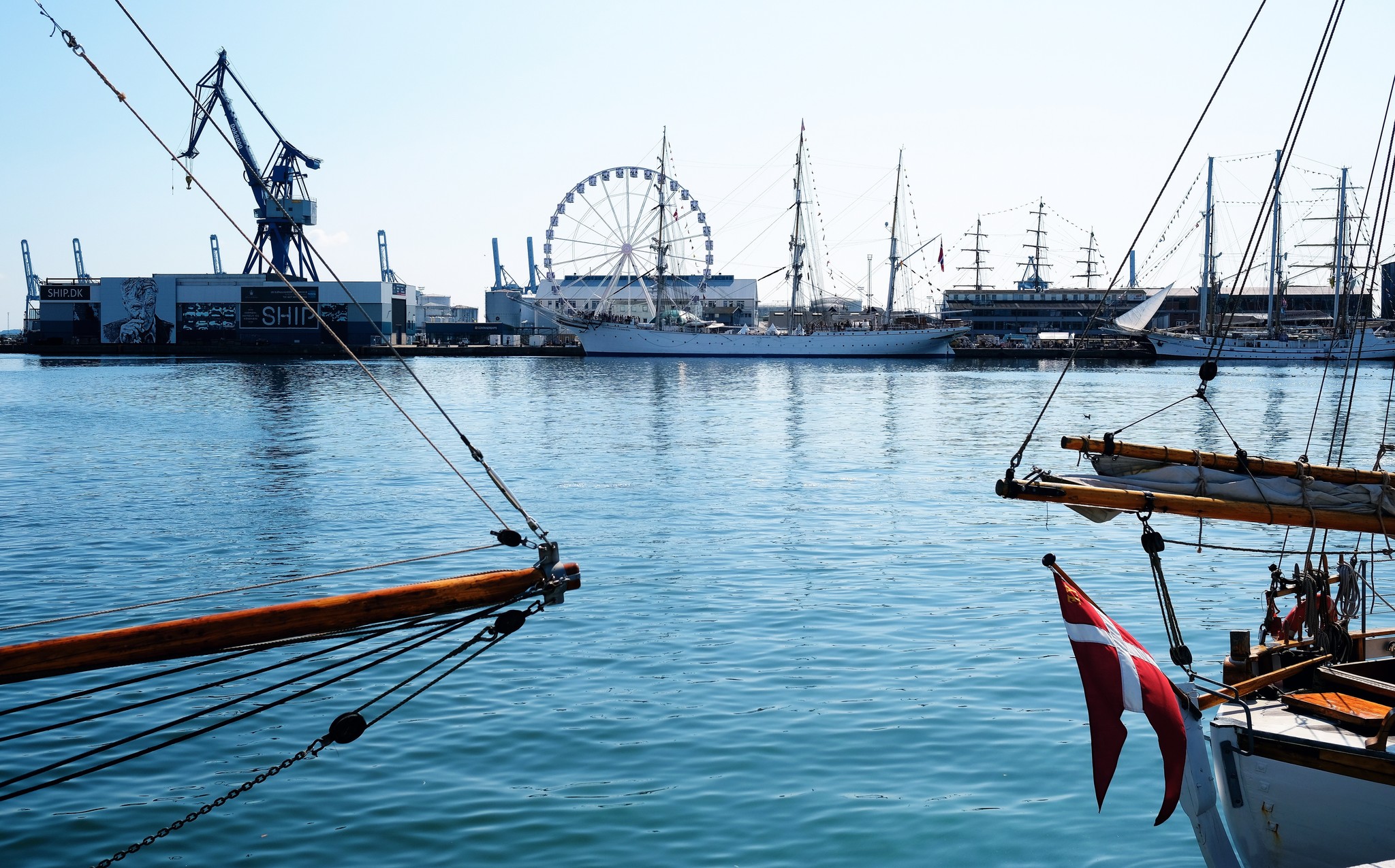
437 345 448 347
417 345 427 347
458 345 468 347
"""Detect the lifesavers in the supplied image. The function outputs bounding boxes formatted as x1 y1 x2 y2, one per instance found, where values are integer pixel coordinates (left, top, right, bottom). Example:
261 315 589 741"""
1274 595 1339 640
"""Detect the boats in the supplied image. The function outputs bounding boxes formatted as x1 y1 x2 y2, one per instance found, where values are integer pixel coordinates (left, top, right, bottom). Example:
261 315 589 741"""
506 118 973 360
1042 552 1395 868
994 431 1395 541
1097 152 1395 361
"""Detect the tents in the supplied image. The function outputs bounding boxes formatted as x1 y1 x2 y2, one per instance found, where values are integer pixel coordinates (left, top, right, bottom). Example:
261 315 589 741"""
794 323 806 335
706 322 725 334
765 323 783 335
737 323 750 334
1036 332 1069 346
687 319 717 326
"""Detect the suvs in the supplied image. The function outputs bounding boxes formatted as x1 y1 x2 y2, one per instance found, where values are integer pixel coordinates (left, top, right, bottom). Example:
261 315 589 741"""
462 339 471 345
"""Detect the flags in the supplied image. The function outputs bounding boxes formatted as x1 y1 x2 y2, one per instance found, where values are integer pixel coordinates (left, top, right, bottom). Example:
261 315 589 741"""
1281 297 1287 315
673 209 678 221
938 238 944 272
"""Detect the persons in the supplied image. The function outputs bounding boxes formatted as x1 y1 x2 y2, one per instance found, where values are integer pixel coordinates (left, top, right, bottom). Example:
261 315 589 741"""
447 342 450 347
417 340 428 347
880 321 951 330
544 338 569 347
433 338 436 347
495 339 498 346
665 316 810 337
506 340 509 347
1296 320 1333 327
457 338 470 347
951 340 1135 348
438 339 440 345
1180 330 1209 340
573 310 640 325
812 319 872 332
1249 342 1253 348
534 342 535 346
520 344 522 347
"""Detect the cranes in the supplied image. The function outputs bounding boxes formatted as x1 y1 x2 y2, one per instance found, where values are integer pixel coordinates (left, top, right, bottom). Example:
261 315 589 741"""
490 236 541 295
72 237 93 283
21 239 46 320
210 234 227 275
172 46 324 284
377 229 406 284
